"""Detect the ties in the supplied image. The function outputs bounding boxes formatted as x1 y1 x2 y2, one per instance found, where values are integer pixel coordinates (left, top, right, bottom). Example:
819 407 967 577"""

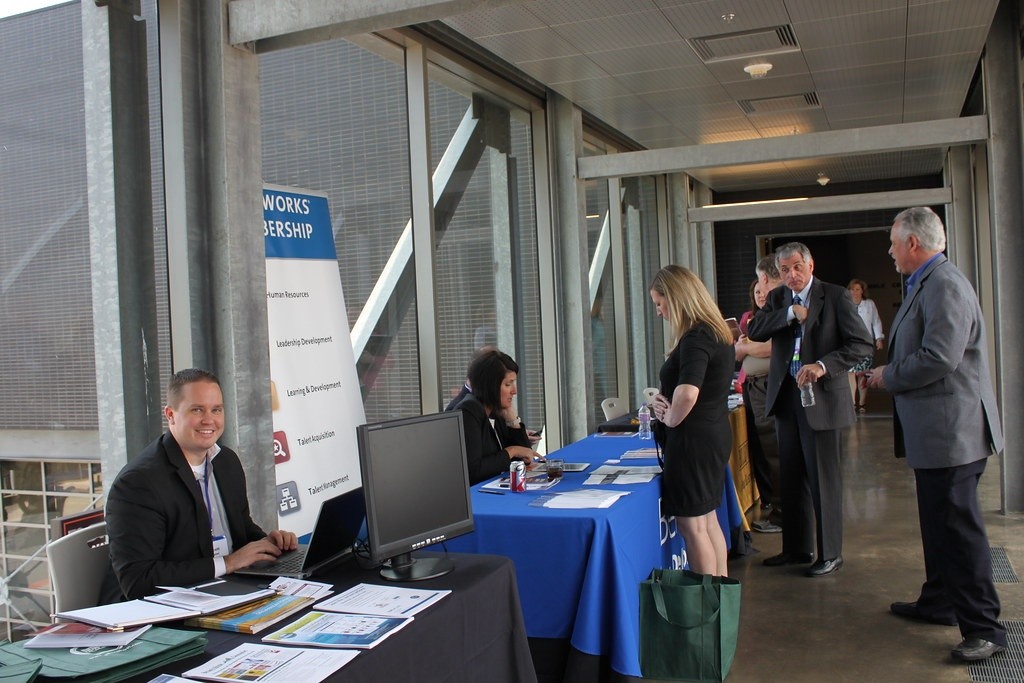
789 295 804 378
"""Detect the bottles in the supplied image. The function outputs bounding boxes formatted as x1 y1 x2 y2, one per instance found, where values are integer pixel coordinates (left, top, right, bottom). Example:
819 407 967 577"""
638 402 651 440
800 377 815 407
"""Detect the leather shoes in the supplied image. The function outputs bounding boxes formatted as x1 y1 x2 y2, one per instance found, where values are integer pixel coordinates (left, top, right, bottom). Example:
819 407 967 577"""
809 554 843 577
951 634 1006 662
890 598 958 624
764 550 814 566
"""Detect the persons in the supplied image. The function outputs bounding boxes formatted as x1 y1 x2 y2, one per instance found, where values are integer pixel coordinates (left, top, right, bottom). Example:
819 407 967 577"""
846 278 885 413
98 368 298 605
735 253 782 532
444 344 546 486
738 280 771 510
650 264 736 577
746 243 873 575
854 207 1008 658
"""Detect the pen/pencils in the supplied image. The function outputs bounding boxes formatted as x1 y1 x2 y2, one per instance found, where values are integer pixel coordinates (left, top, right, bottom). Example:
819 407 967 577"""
478 490 504 495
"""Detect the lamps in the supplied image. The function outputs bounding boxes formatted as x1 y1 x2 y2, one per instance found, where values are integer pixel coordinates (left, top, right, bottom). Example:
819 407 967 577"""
743 58 773 79
816 174 830 185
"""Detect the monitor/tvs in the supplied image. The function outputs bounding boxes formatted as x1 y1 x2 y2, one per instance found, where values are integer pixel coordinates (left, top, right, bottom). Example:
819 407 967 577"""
356 409 474 581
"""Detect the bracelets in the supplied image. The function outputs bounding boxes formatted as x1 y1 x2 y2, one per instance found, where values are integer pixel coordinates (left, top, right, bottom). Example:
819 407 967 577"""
505 416 521 426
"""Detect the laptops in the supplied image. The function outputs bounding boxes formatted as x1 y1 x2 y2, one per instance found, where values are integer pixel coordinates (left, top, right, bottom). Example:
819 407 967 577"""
235 485 366 579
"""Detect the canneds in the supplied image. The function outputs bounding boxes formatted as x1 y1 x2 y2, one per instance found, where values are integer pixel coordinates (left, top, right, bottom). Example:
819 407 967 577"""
509 461 527 494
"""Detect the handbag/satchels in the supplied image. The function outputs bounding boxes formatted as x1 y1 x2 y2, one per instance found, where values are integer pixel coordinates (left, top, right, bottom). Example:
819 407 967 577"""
637 568 741 683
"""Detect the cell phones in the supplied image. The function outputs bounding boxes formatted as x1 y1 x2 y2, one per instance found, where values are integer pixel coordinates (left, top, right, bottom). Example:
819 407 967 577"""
531 429 544 437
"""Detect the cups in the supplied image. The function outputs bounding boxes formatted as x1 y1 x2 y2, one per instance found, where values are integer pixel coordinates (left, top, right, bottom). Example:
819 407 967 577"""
546 459 564 482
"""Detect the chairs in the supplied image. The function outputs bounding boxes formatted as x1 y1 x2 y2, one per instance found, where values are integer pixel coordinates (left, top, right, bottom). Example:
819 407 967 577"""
46 521 112 624
643 388 660 404
601 395 629 422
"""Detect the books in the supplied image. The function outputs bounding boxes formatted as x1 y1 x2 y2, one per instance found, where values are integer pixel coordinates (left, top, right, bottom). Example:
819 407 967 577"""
150 583 452 683
23 622 153 648
50 579 277 628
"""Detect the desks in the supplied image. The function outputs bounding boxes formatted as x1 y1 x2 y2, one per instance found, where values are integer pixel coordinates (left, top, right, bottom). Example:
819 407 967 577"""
345 431 744 683
0 545 539 683
598 393 760 531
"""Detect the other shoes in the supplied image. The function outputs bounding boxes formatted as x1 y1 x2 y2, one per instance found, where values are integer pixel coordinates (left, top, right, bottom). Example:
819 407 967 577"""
859 404 865 412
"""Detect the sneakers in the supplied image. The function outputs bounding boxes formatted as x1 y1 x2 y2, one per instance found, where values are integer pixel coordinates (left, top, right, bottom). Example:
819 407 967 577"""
753 518 780 534
